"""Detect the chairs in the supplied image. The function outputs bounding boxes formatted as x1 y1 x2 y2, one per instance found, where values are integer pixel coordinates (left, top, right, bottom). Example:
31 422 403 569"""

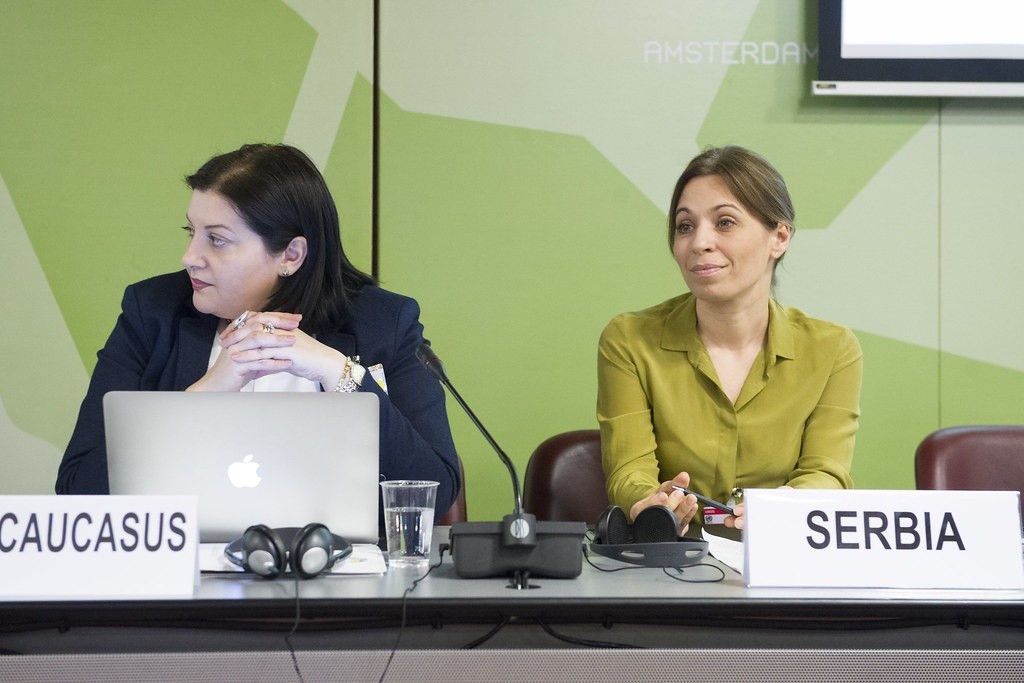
521 430 611 523
915 425 1024 536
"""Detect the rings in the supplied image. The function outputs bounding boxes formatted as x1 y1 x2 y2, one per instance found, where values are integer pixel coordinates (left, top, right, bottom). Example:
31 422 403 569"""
262 322 273 334
233 311 248 329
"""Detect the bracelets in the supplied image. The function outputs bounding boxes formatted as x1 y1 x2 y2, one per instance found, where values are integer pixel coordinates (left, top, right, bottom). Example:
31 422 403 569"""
333 355 360 392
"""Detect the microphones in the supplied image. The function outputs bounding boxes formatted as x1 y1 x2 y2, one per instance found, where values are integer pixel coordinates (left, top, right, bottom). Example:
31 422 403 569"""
414 344 522 512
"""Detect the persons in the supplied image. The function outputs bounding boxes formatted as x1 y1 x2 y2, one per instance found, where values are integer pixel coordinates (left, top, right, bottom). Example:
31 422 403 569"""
55 144 461 519
597 146 863 538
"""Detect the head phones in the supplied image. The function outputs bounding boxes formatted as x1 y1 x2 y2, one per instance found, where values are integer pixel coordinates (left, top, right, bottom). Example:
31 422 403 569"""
224 523 352 579
589 505 709 567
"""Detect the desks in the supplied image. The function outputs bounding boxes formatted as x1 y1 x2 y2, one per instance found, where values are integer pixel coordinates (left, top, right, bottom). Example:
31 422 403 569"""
0 525 1024 683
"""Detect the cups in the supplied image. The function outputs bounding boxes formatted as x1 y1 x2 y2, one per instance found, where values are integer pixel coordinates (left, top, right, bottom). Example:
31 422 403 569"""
379 480 440 567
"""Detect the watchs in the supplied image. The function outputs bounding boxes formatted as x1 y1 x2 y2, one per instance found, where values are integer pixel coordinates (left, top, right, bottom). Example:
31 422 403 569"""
340 364 366 392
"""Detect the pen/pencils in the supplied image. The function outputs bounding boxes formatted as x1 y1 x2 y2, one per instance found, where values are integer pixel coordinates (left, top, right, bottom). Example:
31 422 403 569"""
671 481 735 517
